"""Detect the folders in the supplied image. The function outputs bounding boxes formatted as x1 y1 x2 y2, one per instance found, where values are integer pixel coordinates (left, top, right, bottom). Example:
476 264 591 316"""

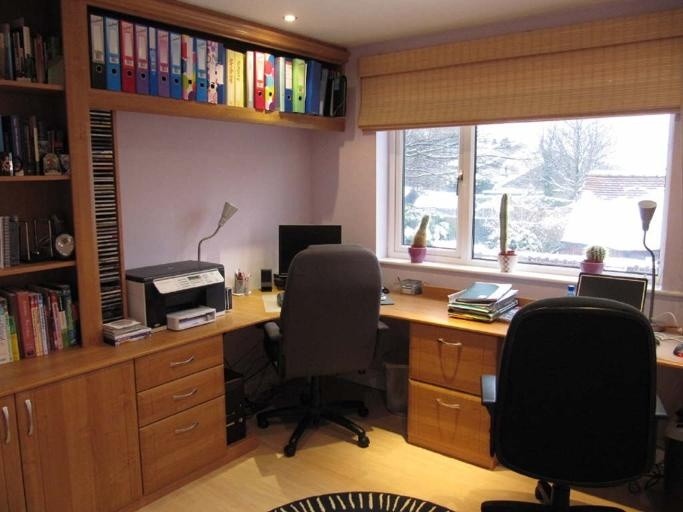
447 281 520 322
88 6 329 117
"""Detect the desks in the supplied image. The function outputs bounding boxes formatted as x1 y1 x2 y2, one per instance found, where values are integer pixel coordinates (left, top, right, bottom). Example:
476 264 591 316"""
132 278 683 502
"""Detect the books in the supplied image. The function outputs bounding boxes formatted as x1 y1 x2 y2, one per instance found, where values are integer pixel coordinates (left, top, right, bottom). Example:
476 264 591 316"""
1 17 85 366
448 281 519 323
103 317 152 346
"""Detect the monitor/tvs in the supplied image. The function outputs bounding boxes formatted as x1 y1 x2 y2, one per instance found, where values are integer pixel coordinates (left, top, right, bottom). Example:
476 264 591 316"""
576 272 648 312
279 224 342 277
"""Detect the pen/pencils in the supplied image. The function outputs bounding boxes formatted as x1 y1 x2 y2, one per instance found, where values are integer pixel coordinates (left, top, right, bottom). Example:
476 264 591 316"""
234 267 246 293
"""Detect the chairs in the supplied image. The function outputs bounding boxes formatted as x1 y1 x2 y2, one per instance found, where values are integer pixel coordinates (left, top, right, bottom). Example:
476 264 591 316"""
475 292 668 511
250 242 381 460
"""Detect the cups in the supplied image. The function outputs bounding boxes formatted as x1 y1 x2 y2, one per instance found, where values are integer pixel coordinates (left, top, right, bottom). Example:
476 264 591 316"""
0 150 23 177
235 278 247 292
260 267 271 292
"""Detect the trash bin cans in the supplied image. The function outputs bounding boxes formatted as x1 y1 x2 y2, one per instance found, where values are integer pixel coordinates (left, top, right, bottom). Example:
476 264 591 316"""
384 356 409 412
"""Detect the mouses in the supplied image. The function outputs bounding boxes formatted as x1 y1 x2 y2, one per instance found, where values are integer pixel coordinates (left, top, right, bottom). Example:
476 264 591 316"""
674 341 683 356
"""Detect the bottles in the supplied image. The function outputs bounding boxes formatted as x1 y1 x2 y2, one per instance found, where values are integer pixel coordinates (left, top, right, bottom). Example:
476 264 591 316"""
566 284 575 296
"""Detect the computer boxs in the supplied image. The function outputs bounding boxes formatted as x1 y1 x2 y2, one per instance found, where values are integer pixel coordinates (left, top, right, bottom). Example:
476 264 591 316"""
224 367 246 445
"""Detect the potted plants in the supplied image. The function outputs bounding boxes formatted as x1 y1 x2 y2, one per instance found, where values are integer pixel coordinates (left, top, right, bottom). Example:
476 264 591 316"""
497 193 519 273
579 245 609 275
407 213 434 264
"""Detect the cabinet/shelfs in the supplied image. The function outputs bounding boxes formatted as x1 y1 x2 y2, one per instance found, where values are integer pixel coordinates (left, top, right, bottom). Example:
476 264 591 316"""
85 0 349 136
0 341 145 512
0 0 103 357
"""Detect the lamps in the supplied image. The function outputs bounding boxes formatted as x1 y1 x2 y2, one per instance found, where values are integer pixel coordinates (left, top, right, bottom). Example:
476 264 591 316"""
636 199 666 331
196 199 240 263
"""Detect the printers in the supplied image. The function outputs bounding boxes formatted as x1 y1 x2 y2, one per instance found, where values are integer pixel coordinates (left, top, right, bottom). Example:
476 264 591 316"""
125 260 226 334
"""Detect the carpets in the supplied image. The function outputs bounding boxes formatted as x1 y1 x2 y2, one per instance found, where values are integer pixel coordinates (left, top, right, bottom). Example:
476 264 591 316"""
266 491 455 511
